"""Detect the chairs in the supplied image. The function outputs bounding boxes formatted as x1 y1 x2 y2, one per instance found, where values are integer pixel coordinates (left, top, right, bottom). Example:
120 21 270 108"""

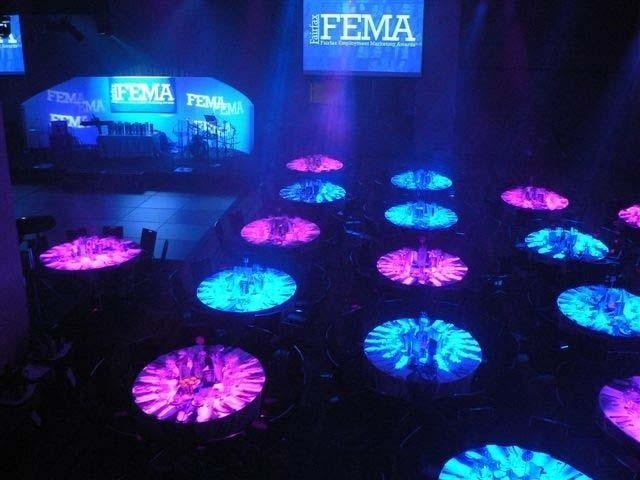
14 153 640 480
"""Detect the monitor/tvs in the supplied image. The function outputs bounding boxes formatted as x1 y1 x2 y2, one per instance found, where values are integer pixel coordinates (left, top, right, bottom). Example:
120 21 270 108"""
301 0 428 80
0 13 28 80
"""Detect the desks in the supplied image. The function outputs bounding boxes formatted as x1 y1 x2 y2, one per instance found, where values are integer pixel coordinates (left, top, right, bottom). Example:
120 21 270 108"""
97 133 161 158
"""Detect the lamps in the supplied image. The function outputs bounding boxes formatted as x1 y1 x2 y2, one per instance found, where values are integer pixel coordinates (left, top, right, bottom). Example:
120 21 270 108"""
33 0 114 42
